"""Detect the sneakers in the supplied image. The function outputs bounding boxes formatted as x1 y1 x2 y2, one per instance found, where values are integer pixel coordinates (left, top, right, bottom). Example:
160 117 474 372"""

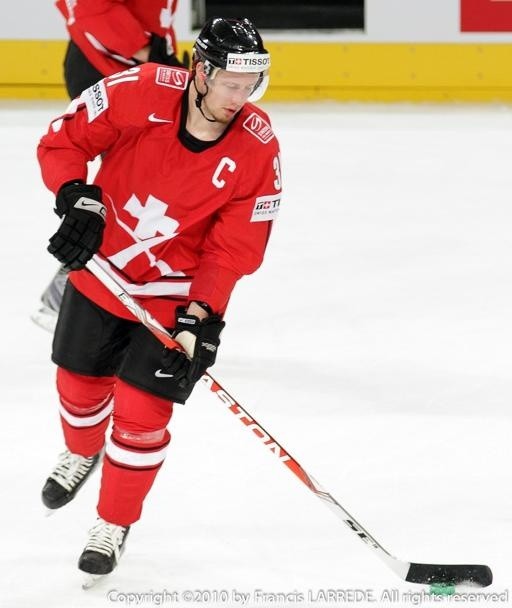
41 271 70 311
42 443 100 510
77 516 130 575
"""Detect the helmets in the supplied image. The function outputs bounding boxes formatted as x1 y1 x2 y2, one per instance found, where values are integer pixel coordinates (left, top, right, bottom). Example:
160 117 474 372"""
193 17 271 98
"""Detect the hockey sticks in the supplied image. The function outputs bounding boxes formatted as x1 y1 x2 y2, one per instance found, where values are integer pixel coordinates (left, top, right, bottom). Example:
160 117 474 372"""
86 260 493 587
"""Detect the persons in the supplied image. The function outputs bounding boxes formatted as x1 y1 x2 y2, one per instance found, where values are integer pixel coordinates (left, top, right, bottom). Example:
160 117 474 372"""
24 2 191 305
36 18 284 575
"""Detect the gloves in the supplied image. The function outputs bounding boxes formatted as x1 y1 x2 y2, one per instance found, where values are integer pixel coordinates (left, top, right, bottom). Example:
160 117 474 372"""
47 186 108 270
159 306 226 399
